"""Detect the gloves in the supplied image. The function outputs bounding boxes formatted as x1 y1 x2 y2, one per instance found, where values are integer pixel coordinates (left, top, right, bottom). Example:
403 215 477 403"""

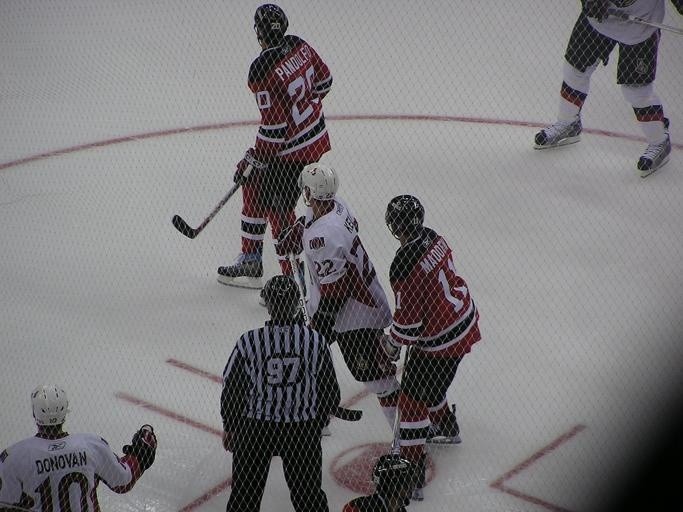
274 216 307 256
233 148 267 185
379 334 402 362
307 313 335 341
581 0 611 24
123 424 157 471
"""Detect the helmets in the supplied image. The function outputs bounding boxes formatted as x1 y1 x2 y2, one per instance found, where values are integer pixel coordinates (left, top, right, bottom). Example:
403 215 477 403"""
371 455 418 490
253 4 289 40
297 163 340 201
385 195 425 236
31 384 68 426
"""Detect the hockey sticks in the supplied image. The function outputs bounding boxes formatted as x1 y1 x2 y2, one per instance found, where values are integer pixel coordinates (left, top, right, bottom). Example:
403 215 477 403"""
171 183 240 240
389 344 410 455
605 11 682 36
288 251 362 422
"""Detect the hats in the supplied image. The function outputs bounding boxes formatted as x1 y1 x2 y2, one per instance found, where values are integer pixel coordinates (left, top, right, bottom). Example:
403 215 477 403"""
260 275 301 307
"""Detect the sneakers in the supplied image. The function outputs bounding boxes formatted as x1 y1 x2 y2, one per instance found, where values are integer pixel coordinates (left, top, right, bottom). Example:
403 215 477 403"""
637 131 671 170
218 253 264 278
427 414 459 439
412 452 427 489
535 113 583 145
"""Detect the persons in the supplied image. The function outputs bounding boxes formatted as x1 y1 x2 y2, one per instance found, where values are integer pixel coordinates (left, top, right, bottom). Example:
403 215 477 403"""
532 0 683 177
377 195 482 501
218 4 334 306
343 454 424 512
209 275 343 512
0 384 157 512
276 163 401 433
